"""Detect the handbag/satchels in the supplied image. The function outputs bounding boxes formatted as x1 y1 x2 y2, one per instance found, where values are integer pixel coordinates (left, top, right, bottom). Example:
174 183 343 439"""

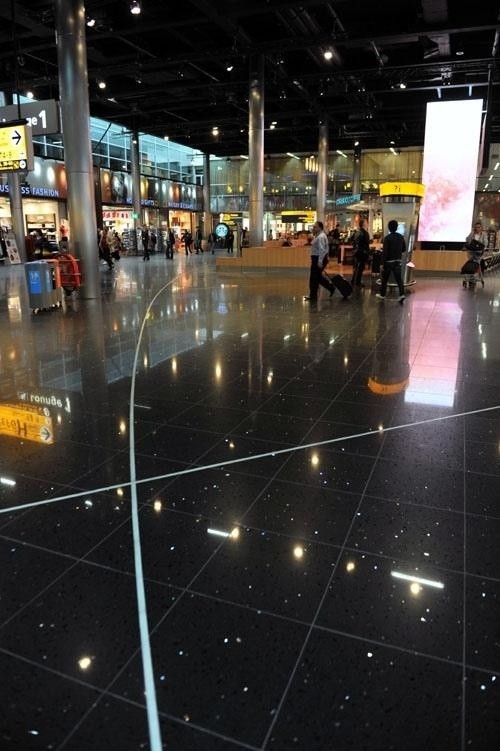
470 239 485 251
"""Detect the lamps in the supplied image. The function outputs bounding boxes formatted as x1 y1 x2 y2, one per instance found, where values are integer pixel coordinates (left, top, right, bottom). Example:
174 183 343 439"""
128 1 143 16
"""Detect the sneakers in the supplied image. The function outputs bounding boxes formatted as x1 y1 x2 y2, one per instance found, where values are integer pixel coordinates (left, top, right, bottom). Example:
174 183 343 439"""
396 295 406 302
375 293 385 299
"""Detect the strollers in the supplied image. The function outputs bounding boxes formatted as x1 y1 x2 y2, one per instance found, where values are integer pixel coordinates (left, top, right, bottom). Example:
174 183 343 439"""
460 248 485 289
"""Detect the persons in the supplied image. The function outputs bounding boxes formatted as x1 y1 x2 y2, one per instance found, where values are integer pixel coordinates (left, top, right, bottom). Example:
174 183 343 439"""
267 223 382 248
303 221 336 300
111 173 127 204
0 224 249 270
464 222 488 287
350 220 369 288
374 220 406 302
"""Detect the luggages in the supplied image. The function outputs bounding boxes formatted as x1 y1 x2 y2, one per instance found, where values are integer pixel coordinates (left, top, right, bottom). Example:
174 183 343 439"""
323 268 354 298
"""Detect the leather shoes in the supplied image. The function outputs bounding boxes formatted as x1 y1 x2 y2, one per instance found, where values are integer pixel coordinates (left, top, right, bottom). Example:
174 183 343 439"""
329 286 336 296
306 297 317 300
